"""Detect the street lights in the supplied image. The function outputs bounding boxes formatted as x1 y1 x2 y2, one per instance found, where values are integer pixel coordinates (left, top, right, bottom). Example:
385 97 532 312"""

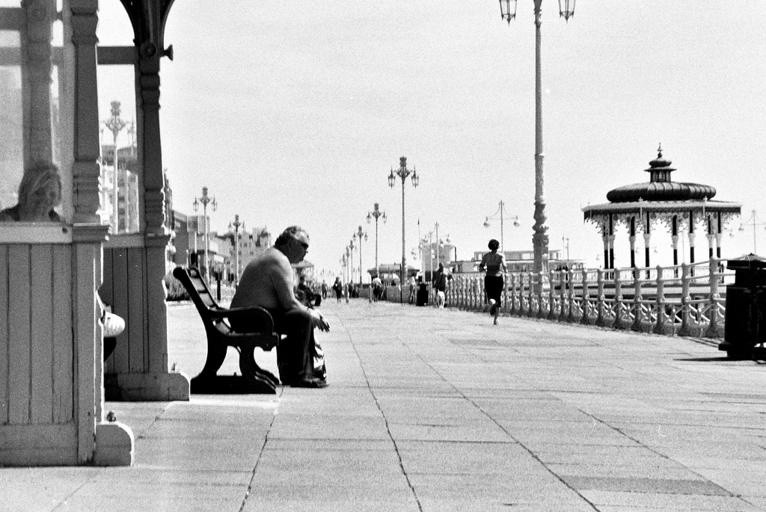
387 155 419 284
227 214 244 287
338 203 387 286
411 221 450 271
483 200 520 254
498 0 576 274
99 101 136 238
192 186 216 288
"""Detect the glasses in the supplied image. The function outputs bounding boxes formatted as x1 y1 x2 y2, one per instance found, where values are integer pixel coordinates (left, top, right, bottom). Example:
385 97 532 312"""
296 240 309 250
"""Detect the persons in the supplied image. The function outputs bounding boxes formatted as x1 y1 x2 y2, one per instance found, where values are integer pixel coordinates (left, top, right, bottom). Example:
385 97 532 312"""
0 161 127 362
480 240 508 326
291 259 456 309
227 226 330 389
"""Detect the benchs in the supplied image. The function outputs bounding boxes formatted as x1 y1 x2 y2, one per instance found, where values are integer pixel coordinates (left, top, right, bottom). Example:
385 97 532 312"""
295 291 314 309
175 267 280 393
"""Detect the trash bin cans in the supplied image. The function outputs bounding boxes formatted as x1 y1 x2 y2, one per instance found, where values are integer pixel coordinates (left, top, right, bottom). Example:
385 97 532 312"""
718 253 766 361
416 283 429 306
